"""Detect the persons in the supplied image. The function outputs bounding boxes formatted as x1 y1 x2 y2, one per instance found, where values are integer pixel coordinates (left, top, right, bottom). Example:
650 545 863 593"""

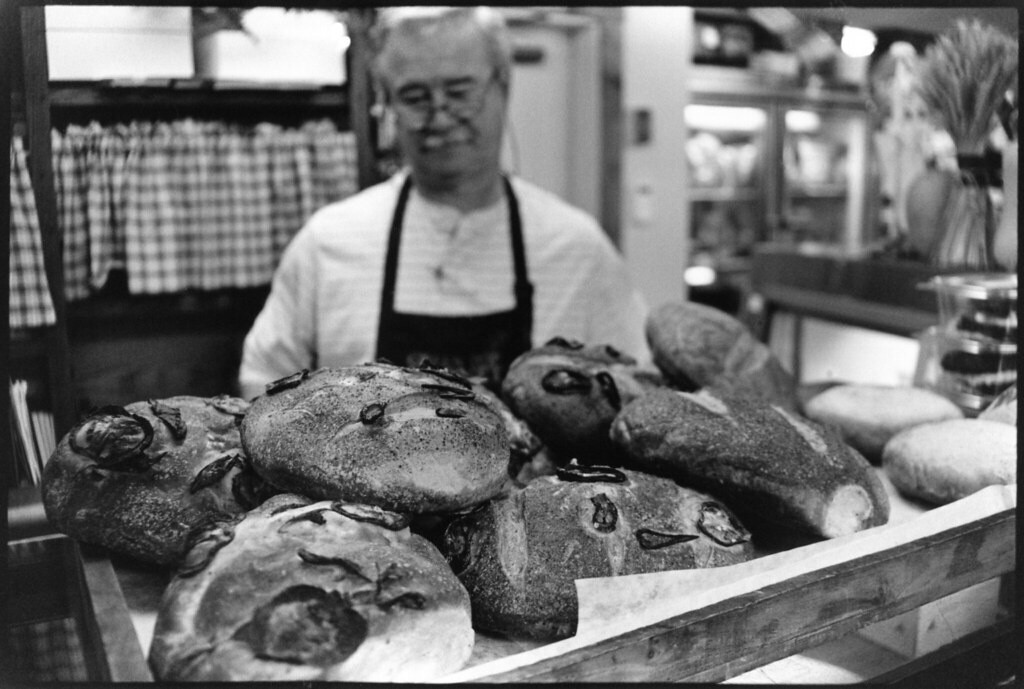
238 5 649 415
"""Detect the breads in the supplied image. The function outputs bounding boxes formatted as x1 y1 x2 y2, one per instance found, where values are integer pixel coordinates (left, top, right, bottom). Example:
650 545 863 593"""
40 397 254 563
884 419 1016 502
610 386 888 540
646 301 807 416
440 460 754 640
148 497 471 681
808 385 961 460
239 362 513 515
504 337 672 457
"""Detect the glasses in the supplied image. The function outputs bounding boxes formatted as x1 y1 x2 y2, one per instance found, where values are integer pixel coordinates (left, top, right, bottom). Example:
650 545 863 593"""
395 72 492 134
